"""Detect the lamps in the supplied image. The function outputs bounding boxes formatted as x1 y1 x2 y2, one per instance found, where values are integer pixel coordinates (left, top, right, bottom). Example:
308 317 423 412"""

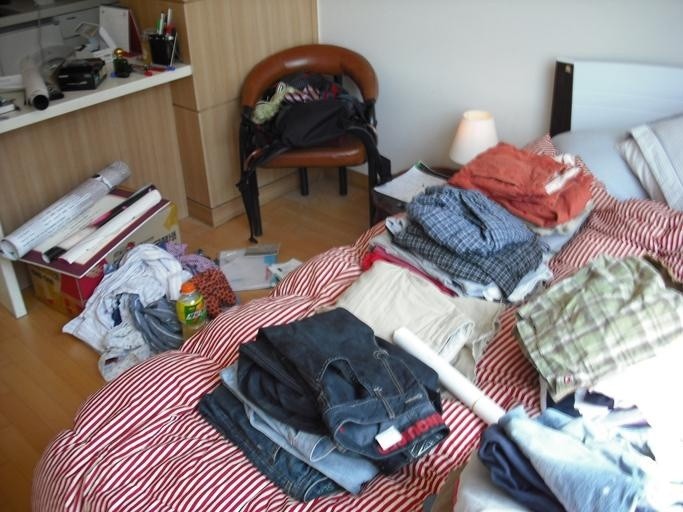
447 110 498 166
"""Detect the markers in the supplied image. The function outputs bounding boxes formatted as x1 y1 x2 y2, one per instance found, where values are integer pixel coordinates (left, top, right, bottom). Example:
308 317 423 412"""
130 66 153 76
145 64 175 70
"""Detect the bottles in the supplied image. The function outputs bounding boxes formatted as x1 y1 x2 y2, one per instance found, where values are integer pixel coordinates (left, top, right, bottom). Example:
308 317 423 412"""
176 282 208 340
112 48 129 78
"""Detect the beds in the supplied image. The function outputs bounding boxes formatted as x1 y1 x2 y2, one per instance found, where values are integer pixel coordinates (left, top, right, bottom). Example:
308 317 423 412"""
33 60 683 512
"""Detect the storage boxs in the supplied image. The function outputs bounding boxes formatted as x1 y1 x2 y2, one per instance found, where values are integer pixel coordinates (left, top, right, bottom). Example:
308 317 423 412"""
27 184 182 319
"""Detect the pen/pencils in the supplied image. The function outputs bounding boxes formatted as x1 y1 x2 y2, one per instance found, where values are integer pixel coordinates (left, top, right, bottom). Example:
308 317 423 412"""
156 7 172 36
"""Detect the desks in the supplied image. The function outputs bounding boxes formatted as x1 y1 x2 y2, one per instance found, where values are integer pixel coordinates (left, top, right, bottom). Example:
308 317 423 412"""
370 167 459 229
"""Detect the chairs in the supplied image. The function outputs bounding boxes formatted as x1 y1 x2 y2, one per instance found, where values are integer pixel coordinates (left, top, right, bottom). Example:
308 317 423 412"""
236 42 379 245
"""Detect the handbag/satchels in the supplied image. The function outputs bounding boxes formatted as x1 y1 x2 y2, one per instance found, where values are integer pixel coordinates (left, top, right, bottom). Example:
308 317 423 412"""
240 96 390 184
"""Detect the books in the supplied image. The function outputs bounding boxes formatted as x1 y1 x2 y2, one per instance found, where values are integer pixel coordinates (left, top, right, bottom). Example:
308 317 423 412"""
216 243 278 291
